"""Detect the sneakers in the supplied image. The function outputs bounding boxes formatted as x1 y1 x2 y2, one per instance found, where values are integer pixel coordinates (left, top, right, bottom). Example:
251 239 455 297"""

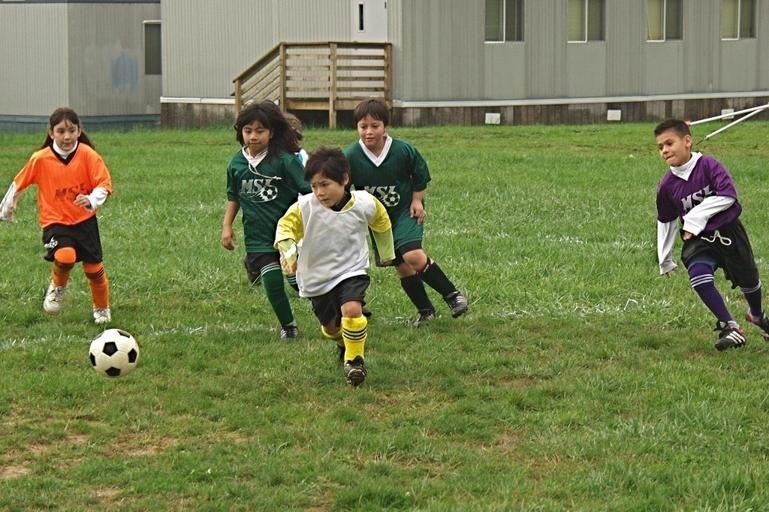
713 319 747 351
343 355 367 387
247 269 262 286
413 308 438 328
93 302 111 324
745 306 769 338
362 307 371 317
337 343 345 362
43 281 65 316
444 290 468 319
280 325 299 340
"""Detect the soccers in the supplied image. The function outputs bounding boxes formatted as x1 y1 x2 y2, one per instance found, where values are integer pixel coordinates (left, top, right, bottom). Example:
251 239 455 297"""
88 329 140 378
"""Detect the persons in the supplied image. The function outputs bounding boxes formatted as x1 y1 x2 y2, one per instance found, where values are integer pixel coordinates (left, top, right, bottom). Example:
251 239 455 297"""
274 145 395 386
0 107 112 323
222 99 372 340
243 112 309 294
344 98 468 328
654 119 769 351
